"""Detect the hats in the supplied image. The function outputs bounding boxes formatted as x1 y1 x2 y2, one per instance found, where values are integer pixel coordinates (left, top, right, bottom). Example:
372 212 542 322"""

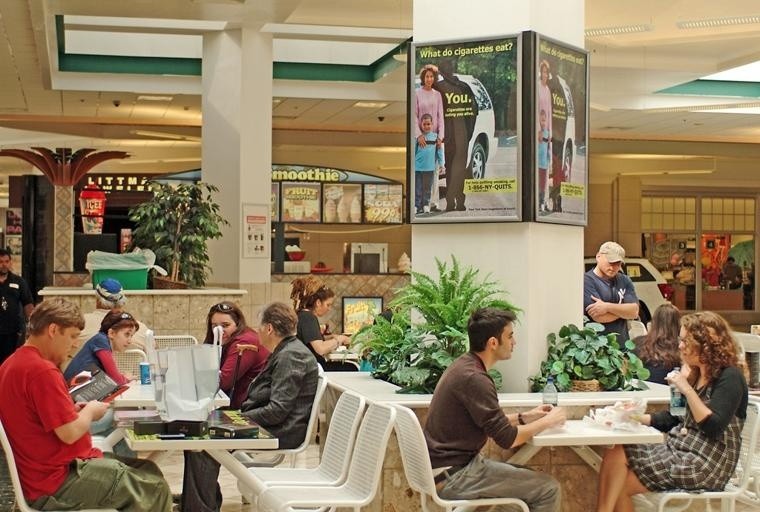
598 241 626 267
96 279 125 302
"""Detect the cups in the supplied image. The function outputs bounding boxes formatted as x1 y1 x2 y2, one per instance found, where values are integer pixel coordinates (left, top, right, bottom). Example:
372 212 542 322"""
79 184 107 235
287 203 315 220
139 362 152 384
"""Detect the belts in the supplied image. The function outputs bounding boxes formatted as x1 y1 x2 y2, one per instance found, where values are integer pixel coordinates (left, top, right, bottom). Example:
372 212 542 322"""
434 463 467 485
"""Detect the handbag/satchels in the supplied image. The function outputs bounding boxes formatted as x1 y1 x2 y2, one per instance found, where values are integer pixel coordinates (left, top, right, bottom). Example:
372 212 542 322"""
145 325 226 425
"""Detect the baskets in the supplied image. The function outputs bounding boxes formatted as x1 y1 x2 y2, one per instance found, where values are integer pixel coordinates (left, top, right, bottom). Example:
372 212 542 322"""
557 353 629 392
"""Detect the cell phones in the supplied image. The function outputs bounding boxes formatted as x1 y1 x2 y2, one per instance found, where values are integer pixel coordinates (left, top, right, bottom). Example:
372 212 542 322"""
157 432 185 438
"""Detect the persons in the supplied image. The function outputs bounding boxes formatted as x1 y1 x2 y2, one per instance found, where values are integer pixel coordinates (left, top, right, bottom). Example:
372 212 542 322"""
537 60 568 213
423 308 566 512
202 300 272 411
584 241 639 356
598 310 749 512
290 274 358 372
173 303 318 512
701 256 742 289
0 248 39 365
415 59 478 213
0 298 173 512
57 278 155 372
627 305 683 383
676 261 696 310
63 308 139 459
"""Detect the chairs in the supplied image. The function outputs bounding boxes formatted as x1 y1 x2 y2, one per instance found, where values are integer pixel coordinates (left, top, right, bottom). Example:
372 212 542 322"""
0 421 118 512
1 309 759 410
391 404 530 512
254 400 397 512
237 390 365 506
642 395 758 511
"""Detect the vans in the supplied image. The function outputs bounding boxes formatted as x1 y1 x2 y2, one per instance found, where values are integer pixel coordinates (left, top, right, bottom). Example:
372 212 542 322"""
538 73 577 185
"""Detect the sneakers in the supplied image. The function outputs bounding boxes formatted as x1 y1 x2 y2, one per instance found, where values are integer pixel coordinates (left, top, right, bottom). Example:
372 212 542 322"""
430 205 442 212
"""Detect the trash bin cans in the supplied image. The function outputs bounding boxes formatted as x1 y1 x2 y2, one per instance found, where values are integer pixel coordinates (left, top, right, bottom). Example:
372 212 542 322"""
92 253 148 289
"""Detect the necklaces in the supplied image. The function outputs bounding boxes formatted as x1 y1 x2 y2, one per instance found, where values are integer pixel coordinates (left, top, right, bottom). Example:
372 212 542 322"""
681 383 707 435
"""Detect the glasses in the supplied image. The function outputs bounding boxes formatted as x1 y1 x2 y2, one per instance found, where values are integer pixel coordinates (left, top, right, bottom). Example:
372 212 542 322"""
105 312 132 330
209 303 234 313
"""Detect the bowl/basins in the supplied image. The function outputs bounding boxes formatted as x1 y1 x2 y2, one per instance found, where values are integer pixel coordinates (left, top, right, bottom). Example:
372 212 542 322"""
287 252 305 261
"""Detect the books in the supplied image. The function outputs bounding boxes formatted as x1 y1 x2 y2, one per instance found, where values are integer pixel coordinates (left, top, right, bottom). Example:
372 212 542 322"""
66 363 129 407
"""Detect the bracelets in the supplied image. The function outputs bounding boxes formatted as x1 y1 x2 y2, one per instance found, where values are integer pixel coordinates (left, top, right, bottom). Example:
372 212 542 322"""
684 387 694 394
518 413 526 425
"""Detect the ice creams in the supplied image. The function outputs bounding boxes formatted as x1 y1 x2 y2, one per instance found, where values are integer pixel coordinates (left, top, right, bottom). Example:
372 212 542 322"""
325 195 361 223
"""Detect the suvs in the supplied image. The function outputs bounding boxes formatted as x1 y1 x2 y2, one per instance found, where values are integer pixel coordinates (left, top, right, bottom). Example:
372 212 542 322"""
584 255 672 328
414 72 498 182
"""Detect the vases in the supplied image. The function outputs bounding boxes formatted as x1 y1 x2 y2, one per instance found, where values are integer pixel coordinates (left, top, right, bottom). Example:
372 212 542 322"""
128 178 229 294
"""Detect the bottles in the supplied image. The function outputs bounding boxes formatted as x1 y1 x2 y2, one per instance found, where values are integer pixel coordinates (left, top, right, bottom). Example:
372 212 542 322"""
668 367 689 417
542 377 559 407
